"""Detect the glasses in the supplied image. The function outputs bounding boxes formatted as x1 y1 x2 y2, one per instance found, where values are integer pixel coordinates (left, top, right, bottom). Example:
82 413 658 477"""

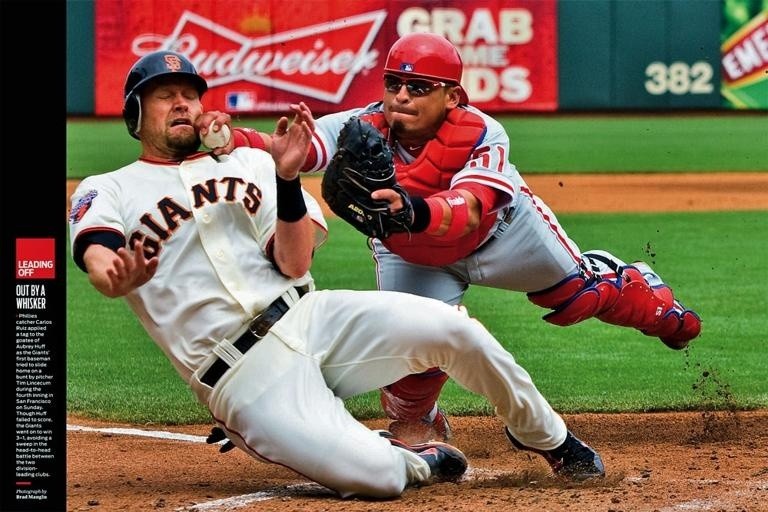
382 73 457 98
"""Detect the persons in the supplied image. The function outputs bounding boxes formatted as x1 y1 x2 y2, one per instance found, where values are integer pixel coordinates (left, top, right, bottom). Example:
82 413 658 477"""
195 31 703 443
66 50 607 502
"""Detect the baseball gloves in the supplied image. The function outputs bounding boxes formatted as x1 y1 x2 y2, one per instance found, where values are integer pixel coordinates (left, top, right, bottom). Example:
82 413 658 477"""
321 117 413 238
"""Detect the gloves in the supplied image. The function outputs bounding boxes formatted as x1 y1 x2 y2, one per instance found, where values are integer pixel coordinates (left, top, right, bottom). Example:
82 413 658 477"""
205 425 238 455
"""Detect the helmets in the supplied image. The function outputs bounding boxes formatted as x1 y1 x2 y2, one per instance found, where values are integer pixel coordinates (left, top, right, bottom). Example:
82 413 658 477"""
384 31 470 106
122 50 209 141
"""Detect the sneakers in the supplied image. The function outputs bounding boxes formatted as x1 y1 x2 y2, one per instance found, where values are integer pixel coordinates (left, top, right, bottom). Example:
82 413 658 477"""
369 427 470 486
501 423 607 482
386 412 453 449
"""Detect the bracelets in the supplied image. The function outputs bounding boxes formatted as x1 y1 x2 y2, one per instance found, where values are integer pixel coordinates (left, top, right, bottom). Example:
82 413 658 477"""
424 196 444 236
409 195 431 233
273 170 308 223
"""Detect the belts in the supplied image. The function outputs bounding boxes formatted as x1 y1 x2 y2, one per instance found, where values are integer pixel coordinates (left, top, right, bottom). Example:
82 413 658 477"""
198 281 312 387
462 206 514 260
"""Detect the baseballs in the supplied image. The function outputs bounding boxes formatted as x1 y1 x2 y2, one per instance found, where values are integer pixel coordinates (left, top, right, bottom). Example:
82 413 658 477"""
200 120 231 147
